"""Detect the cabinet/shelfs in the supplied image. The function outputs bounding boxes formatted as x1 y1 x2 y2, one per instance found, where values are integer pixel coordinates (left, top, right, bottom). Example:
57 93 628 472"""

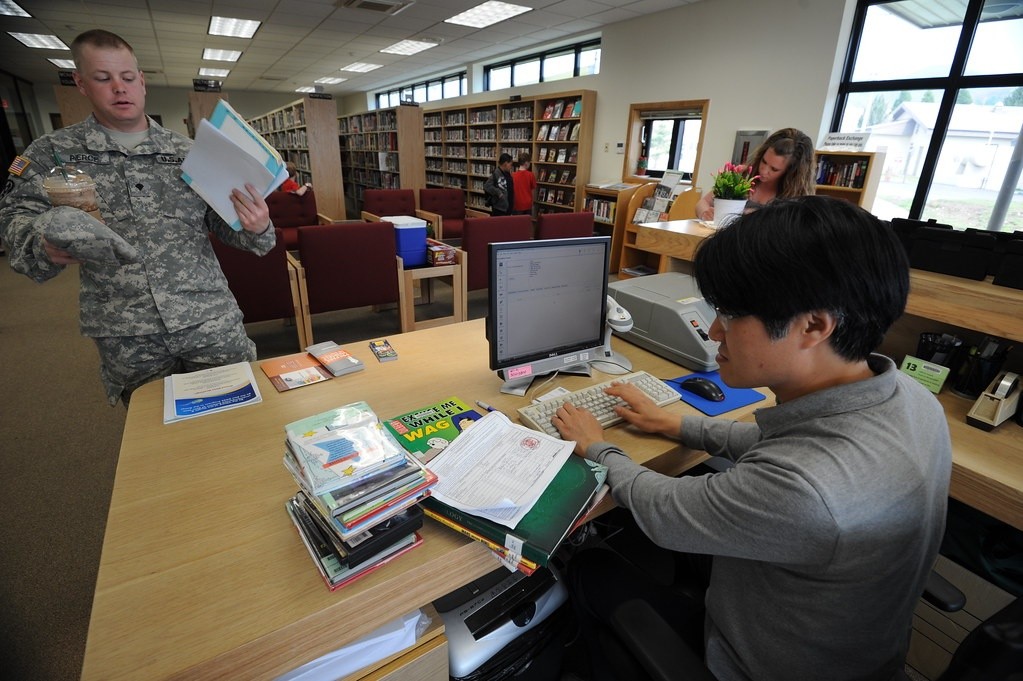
186 91 229 140
583 182 703 279
813 150 887 215
424 89 597 217
54 83 95 128
245 97 346 219
338 106 426 218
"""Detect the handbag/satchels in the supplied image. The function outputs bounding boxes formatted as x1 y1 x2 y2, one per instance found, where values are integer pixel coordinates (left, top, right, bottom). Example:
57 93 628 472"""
484 173 500 207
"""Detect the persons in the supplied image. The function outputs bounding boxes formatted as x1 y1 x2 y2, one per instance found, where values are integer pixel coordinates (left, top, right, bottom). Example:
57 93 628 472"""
695 127 815 221
0 28 276 412
550 195 952 681
280 166 312 196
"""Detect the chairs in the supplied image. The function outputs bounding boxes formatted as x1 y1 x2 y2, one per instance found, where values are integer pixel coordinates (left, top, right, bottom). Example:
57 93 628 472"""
361 189 490 247
265 191 333 250
427 212 594 322
566 546 1018 681
209 222 408 353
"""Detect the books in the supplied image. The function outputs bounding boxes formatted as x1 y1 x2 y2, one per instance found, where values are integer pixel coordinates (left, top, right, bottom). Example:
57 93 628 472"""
369 338 398 362
416 451 610 576
631 184 678 225
538 189 575 207
500 106 532 173
248 107 311 185
582 199 616 223
339 109 399 201
586 179 643 192
283 400 439 593
425 109 497 209
163 361 262 426
539 147 578 163
383 394 483 463
543 97 582 119
815 155 868 188
537 122 581 141
538 170 576 186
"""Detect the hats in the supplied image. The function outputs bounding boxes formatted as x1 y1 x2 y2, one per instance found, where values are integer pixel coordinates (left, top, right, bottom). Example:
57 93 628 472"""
31 206 136 271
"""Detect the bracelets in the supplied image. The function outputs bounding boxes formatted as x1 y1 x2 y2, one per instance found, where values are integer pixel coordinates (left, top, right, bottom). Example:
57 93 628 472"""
305 183 308 188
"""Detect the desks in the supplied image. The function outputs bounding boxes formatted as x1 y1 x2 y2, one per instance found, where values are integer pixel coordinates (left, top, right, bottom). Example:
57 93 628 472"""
404 263 463 332
80 219 1023 681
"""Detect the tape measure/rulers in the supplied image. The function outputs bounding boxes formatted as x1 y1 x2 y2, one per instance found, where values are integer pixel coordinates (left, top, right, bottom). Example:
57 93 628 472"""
981 342 999 358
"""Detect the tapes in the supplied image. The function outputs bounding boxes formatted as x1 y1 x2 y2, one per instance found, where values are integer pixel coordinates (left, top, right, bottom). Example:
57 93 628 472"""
990 371 1019 401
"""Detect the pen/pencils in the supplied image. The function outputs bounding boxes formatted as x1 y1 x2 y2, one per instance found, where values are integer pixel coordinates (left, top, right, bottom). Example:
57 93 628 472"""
474 400 512 420
1000 345 1014 357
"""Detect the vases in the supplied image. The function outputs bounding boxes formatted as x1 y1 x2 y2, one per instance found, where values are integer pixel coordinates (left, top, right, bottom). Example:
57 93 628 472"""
637 168 646 175
714 197 747 229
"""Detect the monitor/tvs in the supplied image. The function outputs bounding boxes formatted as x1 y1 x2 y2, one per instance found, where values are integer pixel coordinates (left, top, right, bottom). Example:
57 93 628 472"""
484 234 611 396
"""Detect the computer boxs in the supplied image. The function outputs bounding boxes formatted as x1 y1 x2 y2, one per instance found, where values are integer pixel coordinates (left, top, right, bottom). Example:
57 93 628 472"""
431 558 571 681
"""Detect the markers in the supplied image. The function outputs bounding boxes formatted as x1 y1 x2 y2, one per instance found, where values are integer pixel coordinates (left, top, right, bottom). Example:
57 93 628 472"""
959 346 977 375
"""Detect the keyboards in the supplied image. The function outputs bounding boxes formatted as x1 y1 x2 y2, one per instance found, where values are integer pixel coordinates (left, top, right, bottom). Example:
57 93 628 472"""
517 370 682 440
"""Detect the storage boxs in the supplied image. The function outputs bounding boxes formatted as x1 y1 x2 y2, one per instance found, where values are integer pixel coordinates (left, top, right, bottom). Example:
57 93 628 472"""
379 216 427 269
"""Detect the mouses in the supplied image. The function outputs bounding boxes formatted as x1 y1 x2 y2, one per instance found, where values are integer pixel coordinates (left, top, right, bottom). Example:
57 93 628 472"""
680 377 728 402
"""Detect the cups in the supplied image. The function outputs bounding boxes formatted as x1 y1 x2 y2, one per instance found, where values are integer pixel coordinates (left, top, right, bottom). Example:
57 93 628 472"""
43 165 101 224
915 332 964 367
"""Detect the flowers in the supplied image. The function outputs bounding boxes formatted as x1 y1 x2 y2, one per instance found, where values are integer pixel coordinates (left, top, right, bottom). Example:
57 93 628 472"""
710 162 762 200
637 157 648 169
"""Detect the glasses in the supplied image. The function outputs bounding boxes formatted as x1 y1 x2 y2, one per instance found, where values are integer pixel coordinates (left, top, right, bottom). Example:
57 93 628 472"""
713 306 762 331
